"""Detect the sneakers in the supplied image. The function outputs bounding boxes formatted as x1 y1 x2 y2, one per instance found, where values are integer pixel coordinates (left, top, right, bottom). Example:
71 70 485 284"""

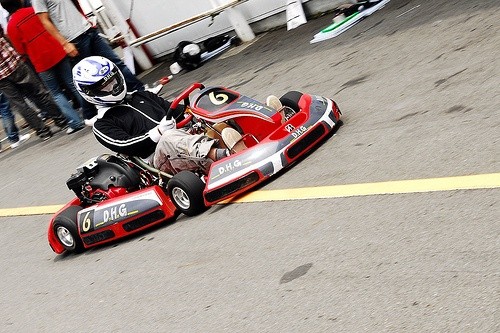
10 134 31 149
142 83 163 94
66 126 85 134
40 133 52 141
266 95 285 125
60 124 69 130
221 127 248 154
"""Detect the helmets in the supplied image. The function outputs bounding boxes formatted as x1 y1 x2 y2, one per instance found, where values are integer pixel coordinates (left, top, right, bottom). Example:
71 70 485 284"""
72 56 127 107
175 41 201 70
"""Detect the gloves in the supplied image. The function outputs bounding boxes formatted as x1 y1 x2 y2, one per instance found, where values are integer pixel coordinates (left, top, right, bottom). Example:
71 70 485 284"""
149 115 176 143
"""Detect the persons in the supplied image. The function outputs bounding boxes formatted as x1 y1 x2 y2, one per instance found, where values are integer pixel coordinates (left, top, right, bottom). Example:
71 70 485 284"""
72 55 285 173
1 0 162 150
